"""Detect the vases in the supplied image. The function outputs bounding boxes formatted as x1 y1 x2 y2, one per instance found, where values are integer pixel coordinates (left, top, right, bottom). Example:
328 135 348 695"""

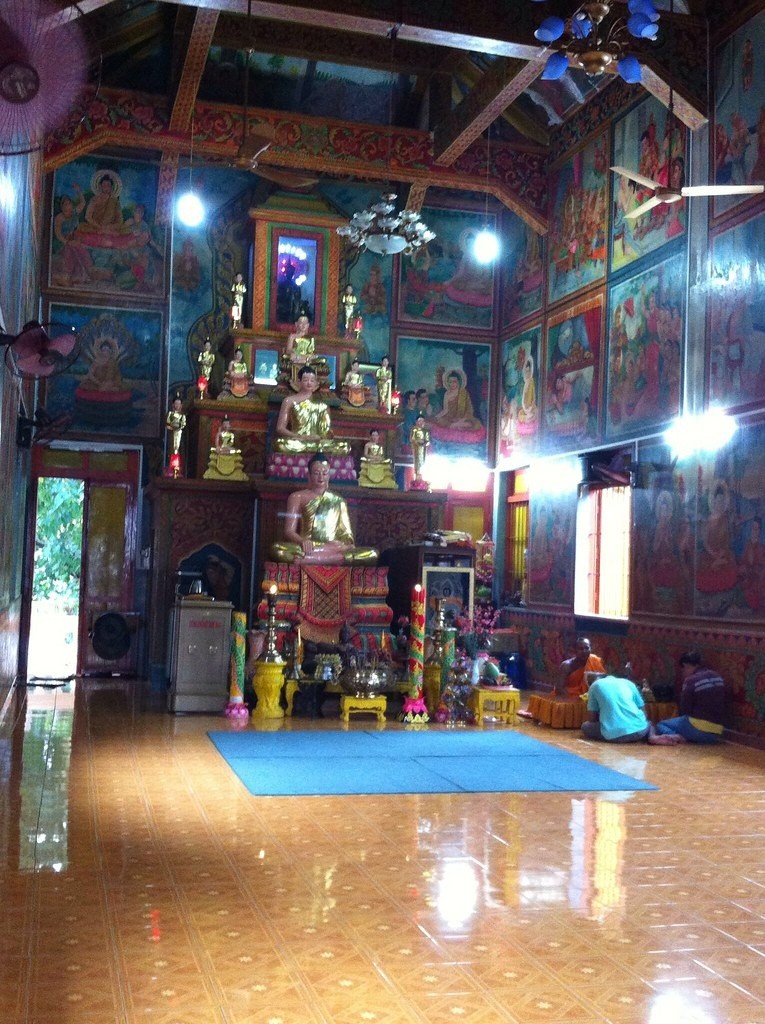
469 660 480 685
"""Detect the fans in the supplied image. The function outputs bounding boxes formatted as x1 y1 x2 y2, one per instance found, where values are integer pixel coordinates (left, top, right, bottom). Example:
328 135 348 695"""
172 0 321 189
0 1 103 157
15 408 81 449
0 320 82 380
607 0 764 220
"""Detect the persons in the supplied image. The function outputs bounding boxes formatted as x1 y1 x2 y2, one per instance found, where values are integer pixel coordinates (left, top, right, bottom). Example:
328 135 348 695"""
198 340 216 382
410 414 431 475
281 312 328 365
210 418 244 454
549 638 607 701
166 396 187 455
581 673 677 746
224 349 253 383
376 357 394 410
358 429 393 463
341 284 357 329
270 452 379 564
230 274 248 329
271 367 351 454
656 651 727 744
342 361 370 391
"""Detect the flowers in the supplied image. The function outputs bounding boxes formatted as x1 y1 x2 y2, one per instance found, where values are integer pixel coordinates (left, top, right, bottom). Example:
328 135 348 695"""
454 604 504 660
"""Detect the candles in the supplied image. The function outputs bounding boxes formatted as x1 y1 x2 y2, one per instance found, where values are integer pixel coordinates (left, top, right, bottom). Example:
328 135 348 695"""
407 585 426 700
229 610 247 704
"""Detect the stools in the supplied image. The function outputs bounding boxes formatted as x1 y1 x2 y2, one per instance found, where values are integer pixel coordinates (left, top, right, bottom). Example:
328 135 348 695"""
469 684 521 728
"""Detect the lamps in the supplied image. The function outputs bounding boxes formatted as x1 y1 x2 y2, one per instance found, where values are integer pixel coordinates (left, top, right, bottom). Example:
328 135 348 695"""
336 24 437 257
533 1 661 86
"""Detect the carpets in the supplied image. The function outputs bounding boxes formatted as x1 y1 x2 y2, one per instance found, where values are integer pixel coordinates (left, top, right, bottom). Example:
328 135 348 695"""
207 728 661 797
